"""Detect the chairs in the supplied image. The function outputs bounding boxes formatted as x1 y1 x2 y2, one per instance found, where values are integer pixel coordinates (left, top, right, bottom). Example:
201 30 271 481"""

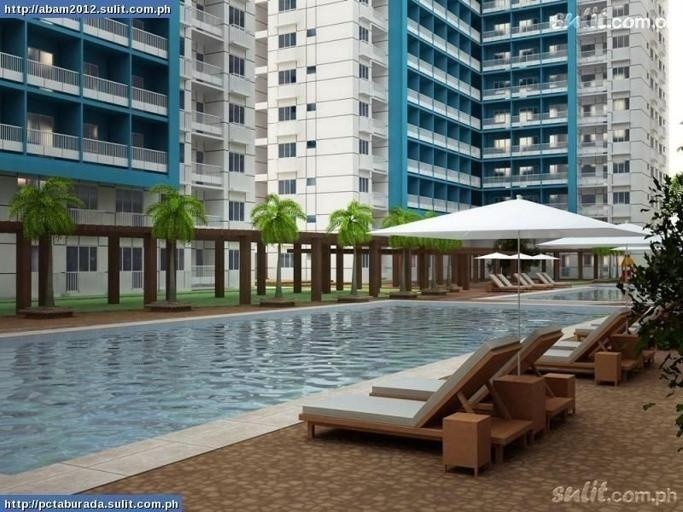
486 272 573 294
298 300 676 478
